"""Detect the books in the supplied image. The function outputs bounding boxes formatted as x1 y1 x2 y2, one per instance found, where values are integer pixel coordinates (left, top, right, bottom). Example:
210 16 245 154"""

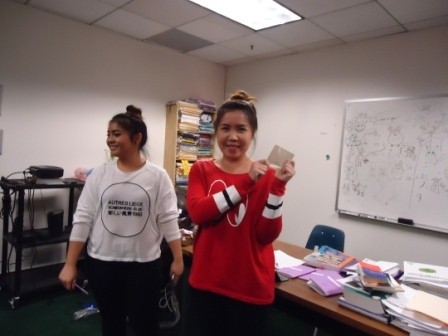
298 269 328 281
276 265 316 279
357 261 392 288
342 275 405 315
347 257 400 279
305 273 344 296
303 245 356 272
176 96 218 176
337 297 396 326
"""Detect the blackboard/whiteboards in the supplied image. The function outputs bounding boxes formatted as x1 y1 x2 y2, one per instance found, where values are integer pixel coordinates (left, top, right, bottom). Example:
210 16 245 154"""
334 93 448 234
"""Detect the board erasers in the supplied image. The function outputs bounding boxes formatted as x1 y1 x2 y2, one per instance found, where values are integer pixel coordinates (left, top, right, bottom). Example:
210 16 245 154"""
397 217 414 225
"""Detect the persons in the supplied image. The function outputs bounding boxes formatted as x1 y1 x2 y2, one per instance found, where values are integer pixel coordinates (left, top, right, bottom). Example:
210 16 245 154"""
186 89 296 336
58 104 184 336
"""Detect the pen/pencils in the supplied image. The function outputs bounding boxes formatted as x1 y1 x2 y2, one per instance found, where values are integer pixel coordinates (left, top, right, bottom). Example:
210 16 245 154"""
74 284 89 294
172 274 175 280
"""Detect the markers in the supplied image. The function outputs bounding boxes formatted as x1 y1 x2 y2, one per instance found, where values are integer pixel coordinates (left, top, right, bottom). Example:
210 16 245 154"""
339 270 355 276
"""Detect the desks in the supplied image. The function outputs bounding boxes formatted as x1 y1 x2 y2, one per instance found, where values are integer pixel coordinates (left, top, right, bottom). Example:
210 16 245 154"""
182 240 409 336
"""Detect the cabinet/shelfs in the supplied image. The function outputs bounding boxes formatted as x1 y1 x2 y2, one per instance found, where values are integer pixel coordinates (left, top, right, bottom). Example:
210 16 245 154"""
0 177 84 309
164 103 217 186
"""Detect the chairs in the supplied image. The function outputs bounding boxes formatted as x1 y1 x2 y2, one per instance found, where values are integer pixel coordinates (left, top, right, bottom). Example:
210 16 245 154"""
305 224 345 253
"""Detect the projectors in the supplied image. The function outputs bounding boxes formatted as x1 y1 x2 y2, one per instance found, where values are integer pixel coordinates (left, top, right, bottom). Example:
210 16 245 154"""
28 165 63 179
